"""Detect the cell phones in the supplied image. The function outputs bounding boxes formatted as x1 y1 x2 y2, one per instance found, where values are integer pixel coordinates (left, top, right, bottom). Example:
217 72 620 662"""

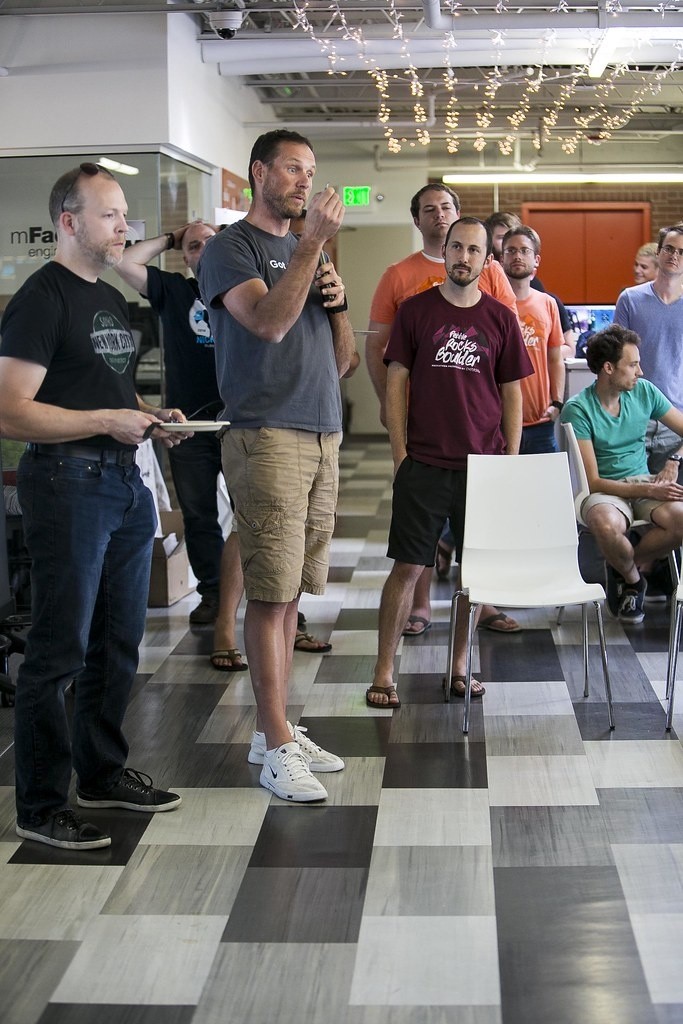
320 251 336 301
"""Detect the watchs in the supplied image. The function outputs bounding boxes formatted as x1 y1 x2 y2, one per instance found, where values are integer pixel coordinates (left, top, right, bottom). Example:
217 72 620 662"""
668 454 683 466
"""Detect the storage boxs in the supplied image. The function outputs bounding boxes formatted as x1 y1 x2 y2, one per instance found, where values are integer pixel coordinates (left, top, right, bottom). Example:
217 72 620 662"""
147 510 189 608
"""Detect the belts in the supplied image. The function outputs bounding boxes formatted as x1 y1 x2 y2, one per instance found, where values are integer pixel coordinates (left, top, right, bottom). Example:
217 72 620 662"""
26 441 138 469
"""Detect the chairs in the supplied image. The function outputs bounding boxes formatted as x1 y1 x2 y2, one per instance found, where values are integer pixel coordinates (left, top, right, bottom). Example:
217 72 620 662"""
553 421 682 626
443 451 615 736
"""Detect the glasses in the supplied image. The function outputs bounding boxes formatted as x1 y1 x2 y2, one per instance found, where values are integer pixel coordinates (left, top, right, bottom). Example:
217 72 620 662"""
501 246 537 257
659 245 682 257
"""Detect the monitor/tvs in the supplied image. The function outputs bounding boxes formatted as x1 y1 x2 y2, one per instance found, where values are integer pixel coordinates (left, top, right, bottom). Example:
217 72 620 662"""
563 304 616 360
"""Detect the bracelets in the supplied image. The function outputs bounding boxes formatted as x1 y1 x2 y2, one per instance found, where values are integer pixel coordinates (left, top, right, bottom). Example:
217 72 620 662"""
322 293 348 314
551 401 564 415
164 231 175 250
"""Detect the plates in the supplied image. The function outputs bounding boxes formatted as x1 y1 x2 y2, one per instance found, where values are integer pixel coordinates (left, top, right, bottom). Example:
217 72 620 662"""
160 420 230 432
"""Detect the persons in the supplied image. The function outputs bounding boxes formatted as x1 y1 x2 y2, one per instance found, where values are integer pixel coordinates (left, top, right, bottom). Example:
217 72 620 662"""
562 323 681 623
632 243 659 285
0 162 197 850
197 127 346 803
547 292 576 358
611 220 683 600
499 225 567 456
366 182 523 636
111 218 248 626
207 207 361 672
436 211 544 578
362 217 537 709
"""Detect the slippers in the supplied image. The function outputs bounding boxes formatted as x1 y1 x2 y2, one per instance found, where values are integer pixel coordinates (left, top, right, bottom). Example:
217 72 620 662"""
401 613 436 638
477 612 521 636
442 672 491 698
291 631 337 656
435 536 453 582
363 679 406 708
206 646 248 674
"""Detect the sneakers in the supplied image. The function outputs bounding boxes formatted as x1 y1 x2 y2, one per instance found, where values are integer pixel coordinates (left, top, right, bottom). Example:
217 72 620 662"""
246 719 348 777
75 767 186 814
15 809 117 851
617 573 649 629
186 592 220 625
258 742 332 806
641 569 669 604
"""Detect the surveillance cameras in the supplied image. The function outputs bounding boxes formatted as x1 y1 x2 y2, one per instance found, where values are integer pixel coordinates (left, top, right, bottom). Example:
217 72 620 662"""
208 12 243 40
